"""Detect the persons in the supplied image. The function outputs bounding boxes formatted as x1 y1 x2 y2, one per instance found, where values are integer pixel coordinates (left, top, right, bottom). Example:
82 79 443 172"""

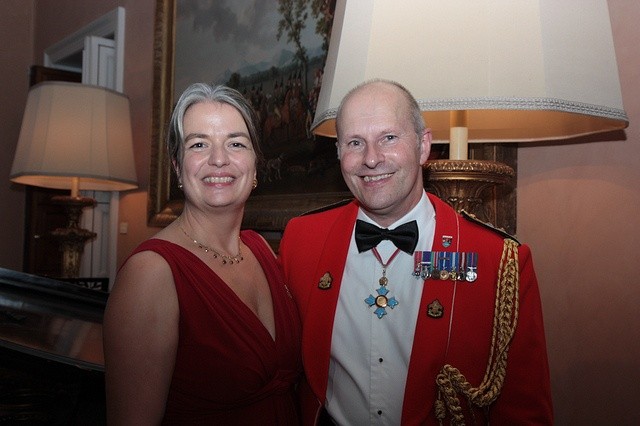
278 78 555 426
101 83 304 426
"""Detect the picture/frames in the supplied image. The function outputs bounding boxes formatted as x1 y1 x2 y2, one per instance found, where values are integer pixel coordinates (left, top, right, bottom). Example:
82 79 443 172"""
145 1 518 235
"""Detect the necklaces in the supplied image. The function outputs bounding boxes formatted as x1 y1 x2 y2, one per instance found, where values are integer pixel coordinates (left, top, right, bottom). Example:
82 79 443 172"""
177 215 245 266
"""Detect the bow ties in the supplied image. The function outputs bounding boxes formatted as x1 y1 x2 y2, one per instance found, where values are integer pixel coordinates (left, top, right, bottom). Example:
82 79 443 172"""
354 219 418 255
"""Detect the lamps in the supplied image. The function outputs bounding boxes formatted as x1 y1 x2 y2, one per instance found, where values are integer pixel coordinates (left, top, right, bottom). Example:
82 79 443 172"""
7 81 140 277
309 0 630 224
